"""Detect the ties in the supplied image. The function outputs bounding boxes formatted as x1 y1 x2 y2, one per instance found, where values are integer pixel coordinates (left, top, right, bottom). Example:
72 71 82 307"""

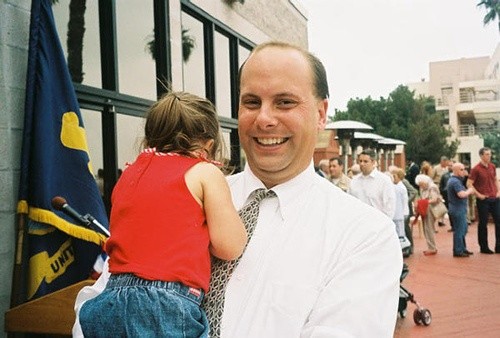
199 189 278 338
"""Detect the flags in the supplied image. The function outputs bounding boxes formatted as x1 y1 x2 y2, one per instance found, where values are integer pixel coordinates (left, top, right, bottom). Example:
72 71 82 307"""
9 0 109 308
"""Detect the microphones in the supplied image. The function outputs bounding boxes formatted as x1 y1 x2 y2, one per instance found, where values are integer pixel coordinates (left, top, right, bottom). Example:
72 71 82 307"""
52 196 93 228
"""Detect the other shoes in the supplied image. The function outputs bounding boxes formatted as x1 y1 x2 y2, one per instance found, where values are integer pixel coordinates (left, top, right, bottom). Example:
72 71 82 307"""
495 247 500 253
480 248 494 254
454 253 470 257
465 250 474 255
423 250 437 255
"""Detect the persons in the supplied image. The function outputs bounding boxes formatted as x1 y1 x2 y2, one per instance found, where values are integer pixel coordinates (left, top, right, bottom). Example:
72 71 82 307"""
316 147 500 258
79 91 247 338
95 168 105 196
72 42 403 338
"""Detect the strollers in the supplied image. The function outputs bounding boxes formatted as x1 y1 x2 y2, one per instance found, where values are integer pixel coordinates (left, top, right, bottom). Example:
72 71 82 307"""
398 235 433 327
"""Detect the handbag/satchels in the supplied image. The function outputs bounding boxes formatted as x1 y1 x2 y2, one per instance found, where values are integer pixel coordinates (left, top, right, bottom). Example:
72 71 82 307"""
430 198 448 219
